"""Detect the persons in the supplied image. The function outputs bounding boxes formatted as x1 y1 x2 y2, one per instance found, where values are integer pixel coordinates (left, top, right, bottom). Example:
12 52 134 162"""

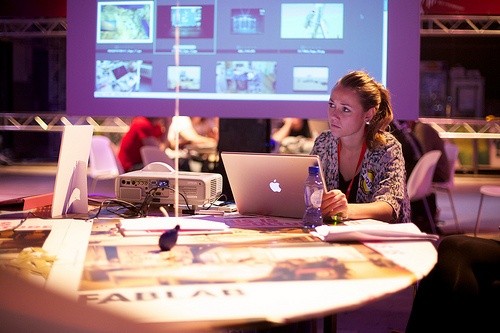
309 71 412 220
118 116 312 170
401 116 448 181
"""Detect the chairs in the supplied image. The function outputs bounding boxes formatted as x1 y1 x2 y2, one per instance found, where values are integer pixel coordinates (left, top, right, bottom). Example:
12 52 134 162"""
407 150 442 235
87 135 123 195
405 233 500 333
413 122 462 233
141 146 176 171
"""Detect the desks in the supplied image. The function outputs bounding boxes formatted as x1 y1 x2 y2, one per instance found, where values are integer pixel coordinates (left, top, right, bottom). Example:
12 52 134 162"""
0 192 439 333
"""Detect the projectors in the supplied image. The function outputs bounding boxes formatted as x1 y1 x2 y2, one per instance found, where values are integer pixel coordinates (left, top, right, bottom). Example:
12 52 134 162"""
115 168 223 205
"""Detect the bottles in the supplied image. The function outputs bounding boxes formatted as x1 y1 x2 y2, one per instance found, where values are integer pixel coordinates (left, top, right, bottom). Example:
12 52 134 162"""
303 167 323 228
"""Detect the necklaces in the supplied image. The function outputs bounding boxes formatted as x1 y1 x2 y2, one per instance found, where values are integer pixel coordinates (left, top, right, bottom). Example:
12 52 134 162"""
326 137 367 196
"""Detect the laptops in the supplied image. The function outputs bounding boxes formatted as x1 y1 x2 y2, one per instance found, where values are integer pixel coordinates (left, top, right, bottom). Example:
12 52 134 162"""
220 151 330 218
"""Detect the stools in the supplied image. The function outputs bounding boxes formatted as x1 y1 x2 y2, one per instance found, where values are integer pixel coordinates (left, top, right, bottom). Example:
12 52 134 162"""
473 187 500 237
